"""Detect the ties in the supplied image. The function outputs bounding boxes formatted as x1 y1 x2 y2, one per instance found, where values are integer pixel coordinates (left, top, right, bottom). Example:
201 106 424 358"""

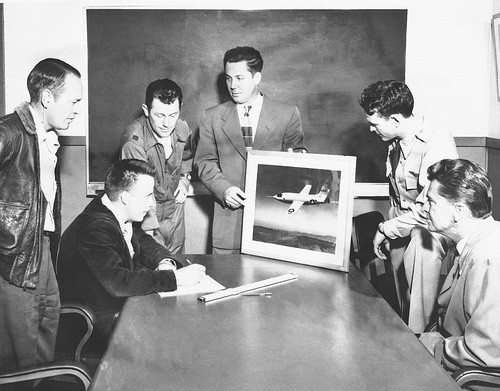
241 105 252 151
389 139 401 205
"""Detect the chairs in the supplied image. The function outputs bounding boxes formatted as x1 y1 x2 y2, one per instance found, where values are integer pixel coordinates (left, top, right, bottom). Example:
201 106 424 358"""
351 210 446 309
0 300 97 391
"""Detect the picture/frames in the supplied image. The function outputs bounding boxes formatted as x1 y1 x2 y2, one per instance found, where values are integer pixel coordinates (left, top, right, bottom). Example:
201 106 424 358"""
240 150 357 273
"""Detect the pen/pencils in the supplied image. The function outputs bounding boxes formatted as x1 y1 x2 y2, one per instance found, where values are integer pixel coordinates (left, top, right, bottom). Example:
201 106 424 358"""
186 259 192 265
243 293 272 296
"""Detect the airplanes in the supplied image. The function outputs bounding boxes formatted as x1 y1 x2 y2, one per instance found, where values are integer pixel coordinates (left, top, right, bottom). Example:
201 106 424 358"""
272 184 331 216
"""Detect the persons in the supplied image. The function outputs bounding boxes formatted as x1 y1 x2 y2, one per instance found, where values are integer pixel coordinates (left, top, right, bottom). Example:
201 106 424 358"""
418 159 500 391
118 78 194 254
57 159 183 353
0 58 83 391
358 79 459 337
192 47 309 254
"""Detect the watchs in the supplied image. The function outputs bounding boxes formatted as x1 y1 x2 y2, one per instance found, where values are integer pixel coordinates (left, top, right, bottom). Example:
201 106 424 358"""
181 174 192 181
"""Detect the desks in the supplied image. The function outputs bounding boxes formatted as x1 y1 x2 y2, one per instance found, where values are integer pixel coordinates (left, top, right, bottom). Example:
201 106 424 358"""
87 254 463 391
